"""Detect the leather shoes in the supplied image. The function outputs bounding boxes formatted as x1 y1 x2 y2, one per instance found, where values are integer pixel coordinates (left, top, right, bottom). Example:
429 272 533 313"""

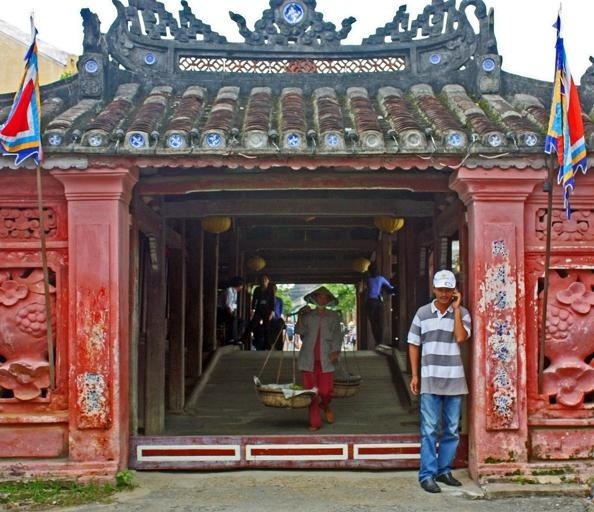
437 473 462 486
422 478 441 492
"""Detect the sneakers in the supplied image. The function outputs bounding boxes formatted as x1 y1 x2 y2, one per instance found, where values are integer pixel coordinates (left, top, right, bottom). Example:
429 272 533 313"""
325 408 334 424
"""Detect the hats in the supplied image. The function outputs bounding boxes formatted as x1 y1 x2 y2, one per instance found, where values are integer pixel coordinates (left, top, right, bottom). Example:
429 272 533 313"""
304 286 338 306
433 269 457 289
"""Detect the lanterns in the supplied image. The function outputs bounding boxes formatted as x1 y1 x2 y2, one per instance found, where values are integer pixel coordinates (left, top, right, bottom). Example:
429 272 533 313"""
374 217 405 234
247 256 266 271
201 216 231 234
352 256 371 274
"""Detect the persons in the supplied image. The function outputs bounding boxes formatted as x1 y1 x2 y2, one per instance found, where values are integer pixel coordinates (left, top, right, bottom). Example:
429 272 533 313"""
273 284 282 321
407 270 471 493
365 262 394 349
296 286 342 432
217 276 245 344
239 273 276 350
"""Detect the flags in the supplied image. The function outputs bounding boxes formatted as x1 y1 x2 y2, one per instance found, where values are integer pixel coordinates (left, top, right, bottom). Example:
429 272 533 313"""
544 7 589 218
0 19 43 170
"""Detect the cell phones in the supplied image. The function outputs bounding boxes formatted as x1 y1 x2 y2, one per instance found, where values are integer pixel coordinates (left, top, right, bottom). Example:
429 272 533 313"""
449 296 454 302
